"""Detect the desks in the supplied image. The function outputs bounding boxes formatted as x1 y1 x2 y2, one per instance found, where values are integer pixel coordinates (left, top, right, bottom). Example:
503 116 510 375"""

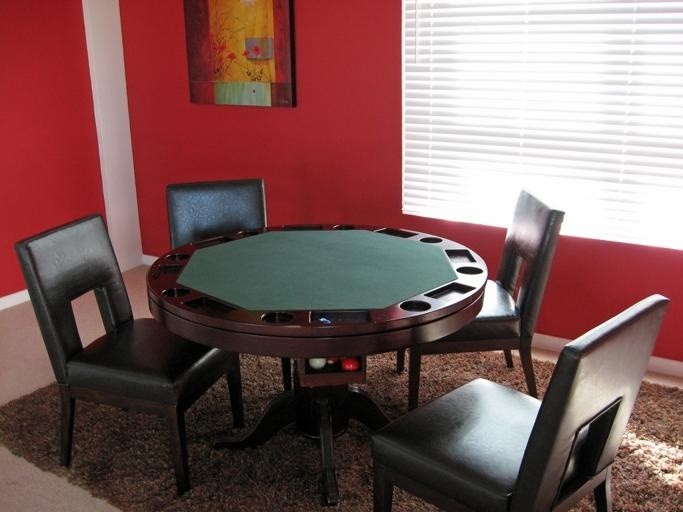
142 220 490 511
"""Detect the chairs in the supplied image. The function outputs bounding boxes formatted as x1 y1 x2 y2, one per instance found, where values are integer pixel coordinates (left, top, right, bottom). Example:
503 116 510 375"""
15 213 246 496
393 188 567 412
369 288 670 512
164 178 272 251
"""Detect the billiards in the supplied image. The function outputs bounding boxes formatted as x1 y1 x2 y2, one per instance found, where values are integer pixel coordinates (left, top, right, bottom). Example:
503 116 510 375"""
308 357 360 372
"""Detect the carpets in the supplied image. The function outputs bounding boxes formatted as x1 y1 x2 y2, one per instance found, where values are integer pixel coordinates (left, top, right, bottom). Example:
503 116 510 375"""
0 353 683 510
